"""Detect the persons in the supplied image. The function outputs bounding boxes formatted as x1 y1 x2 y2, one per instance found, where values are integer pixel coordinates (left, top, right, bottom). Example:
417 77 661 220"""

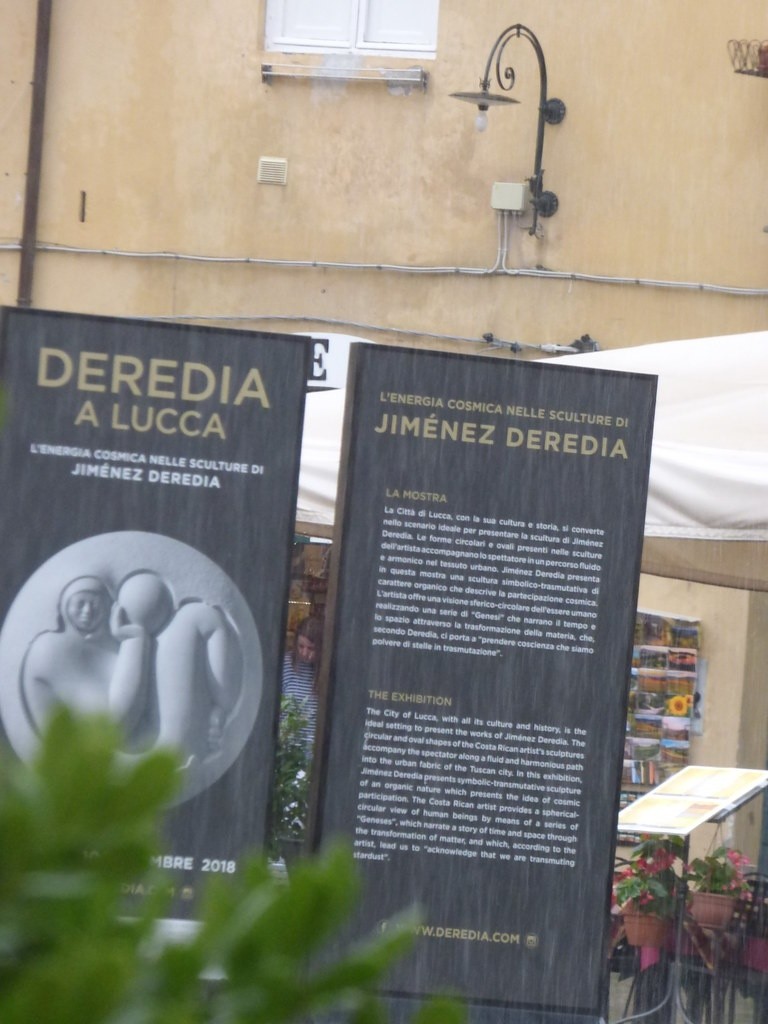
281 617 323 760
693 692 700 718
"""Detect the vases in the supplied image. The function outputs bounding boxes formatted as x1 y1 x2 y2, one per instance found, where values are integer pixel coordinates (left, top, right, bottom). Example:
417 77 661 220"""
619 909 674 946
692 889 739 930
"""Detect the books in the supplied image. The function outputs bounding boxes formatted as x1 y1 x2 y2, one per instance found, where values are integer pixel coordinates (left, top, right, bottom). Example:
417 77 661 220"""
617 612 701 842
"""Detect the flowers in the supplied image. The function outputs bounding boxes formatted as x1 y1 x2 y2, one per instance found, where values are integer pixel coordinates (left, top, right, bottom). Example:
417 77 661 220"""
686 842 759 901
615 837 696 918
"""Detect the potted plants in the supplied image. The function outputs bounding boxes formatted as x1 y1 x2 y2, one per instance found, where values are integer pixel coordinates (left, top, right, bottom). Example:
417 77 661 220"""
622 626 697 791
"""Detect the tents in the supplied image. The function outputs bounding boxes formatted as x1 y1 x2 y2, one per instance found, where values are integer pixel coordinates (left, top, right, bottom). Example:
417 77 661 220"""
296 328 768 595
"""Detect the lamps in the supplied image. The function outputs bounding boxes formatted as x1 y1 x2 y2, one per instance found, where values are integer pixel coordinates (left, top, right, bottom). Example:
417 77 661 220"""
450 22 566 236
